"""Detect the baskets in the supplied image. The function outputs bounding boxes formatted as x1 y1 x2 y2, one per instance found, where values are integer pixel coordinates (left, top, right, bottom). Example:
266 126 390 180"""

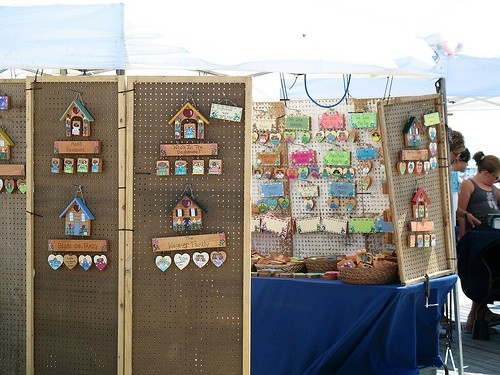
305 257 341 274
336 259 399 284
256 262 306 273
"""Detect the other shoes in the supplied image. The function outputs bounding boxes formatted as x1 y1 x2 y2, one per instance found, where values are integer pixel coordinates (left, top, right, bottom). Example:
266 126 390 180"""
488 315 500 326
466 322 498 334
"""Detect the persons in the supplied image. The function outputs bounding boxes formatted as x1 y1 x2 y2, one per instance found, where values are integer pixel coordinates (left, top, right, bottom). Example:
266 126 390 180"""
438 128 482 338
457 151 500 334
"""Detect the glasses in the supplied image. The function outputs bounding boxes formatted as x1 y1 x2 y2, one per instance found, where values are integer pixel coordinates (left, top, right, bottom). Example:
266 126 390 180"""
490 173 500 182
451 151 462 158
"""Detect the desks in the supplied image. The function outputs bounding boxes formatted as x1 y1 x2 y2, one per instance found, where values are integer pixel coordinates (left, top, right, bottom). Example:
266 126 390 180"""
251 274 458 375
